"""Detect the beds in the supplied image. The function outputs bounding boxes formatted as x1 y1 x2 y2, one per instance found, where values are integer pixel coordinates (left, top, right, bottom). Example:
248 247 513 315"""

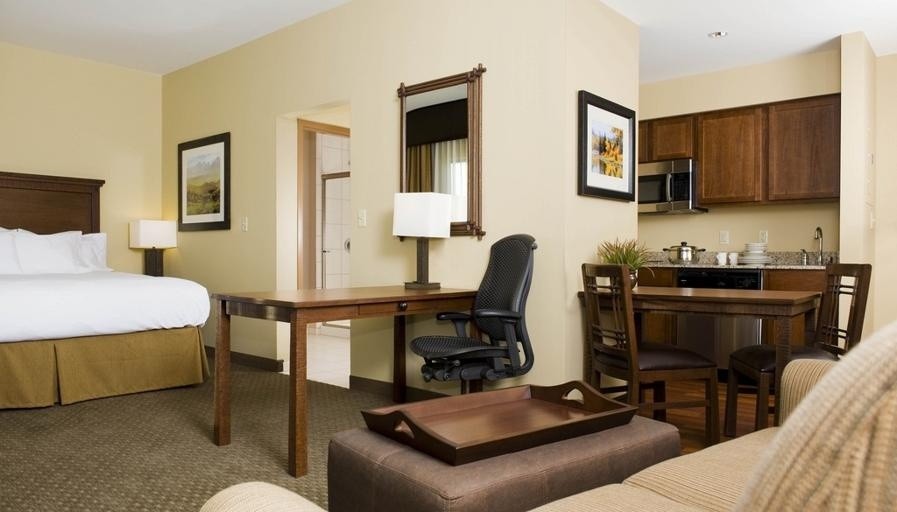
0 165 211 414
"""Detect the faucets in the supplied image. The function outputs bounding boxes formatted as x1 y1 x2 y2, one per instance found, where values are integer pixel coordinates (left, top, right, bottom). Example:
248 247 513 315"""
815 226 823 266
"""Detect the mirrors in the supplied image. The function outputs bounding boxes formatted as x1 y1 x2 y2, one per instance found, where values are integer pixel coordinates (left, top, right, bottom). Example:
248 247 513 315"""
395 64 487 242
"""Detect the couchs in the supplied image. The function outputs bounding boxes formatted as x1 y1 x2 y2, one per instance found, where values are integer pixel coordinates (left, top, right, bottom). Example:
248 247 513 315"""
326 401 682 512
189 309 897 512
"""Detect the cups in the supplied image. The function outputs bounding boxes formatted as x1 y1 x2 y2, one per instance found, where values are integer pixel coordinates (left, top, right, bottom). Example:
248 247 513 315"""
728 252 738 265
717 253 727 265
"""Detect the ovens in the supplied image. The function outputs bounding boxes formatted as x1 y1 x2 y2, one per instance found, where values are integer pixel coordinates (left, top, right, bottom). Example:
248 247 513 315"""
673 268 763 371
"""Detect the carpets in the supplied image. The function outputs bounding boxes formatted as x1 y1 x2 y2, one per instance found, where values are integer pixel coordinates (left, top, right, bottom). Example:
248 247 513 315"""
0 342 432 512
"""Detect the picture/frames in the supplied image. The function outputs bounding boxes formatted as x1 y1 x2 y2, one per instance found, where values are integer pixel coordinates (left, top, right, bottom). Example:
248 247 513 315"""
575 90 638 202
176 131 229 234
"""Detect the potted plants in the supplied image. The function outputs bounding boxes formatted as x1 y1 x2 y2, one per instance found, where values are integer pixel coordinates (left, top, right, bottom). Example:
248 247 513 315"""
596 237 654 288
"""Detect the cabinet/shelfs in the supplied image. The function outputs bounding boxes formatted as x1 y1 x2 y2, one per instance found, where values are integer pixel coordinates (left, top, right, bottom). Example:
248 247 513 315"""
632 266 674 347
635 112 692 161
761 268 839 354
665 306 761 380
692 86 840 208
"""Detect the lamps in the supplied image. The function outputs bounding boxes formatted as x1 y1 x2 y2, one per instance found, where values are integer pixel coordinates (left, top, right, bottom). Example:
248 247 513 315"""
391 189 453 292
129 216 179 275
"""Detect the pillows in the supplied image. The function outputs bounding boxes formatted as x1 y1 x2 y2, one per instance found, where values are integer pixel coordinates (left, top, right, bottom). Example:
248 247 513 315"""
13 229 92 273
71 230 114 274
0 226 25 278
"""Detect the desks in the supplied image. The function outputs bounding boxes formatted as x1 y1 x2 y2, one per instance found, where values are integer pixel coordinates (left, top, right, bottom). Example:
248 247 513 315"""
209 281 489 479
580 284 824 437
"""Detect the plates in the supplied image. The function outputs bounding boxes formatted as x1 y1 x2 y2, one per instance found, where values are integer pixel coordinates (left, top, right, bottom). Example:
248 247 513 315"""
743 242 769 252
736 252 776 266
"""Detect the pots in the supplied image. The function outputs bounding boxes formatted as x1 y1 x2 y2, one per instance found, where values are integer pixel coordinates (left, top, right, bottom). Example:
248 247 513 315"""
661 241 706 264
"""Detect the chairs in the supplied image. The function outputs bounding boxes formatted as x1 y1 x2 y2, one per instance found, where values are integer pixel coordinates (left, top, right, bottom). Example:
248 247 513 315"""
578 258 722 444
722 251 872 438
410 232 538 394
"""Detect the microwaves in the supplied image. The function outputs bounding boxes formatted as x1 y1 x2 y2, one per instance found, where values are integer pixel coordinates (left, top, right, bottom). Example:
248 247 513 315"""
636 157 707 214
